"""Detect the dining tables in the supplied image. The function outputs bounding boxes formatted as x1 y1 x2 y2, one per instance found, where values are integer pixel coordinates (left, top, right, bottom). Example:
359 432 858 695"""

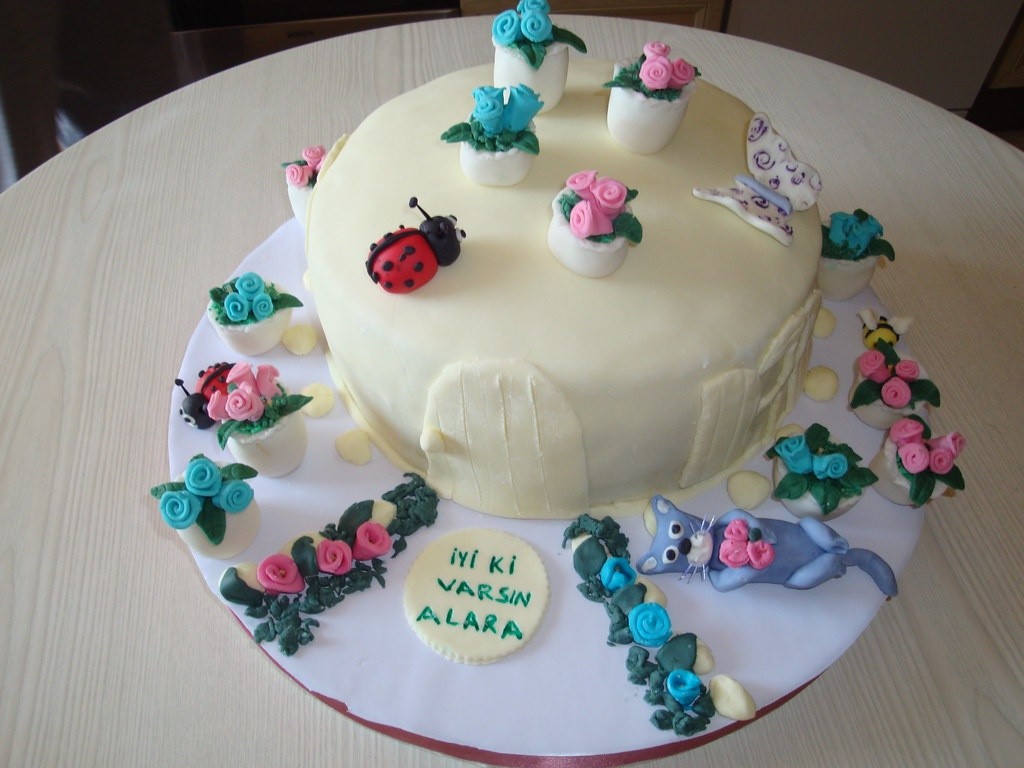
0 13 1024 768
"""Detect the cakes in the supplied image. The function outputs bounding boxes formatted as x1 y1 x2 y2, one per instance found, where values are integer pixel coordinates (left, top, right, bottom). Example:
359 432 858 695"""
160 0 964 735
